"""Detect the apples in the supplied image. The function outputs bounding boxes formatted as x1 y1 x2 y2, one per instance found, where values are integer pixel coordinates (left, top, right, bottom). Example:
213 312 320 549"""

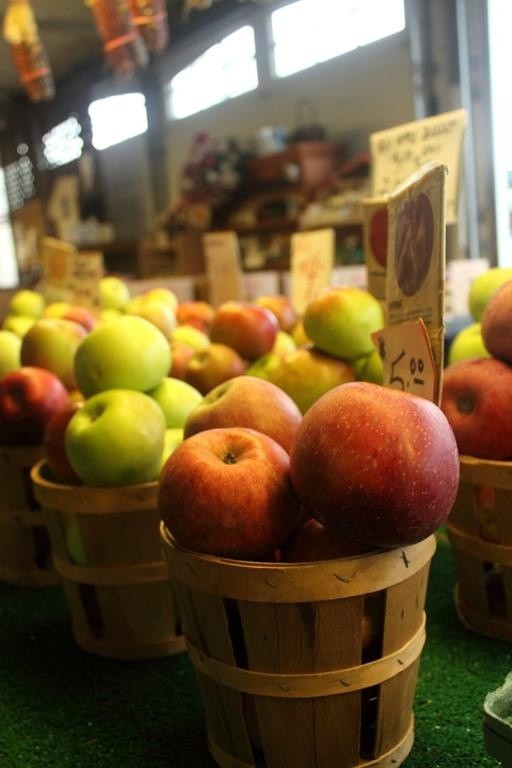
1 266 461 656
429 268 512 463
